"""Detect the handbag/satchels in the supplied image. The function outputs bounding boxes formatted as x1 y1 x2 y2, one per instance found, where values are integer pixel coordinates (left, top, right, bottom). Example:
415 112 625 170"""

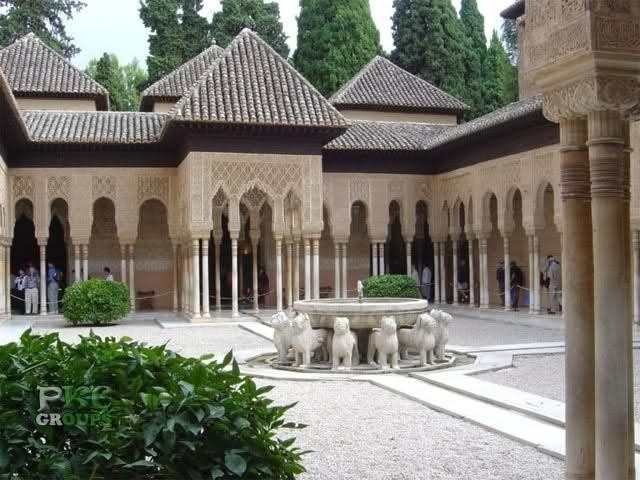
53 271 63 282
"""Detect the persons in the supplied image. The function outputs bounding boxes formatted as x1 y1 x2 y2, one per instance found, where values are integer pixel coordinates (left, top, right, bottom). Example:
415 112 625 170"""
422 264 433 301
511 261 523 311
258 266 270 307
412 264 422 298
14 261 63 316
542 255 562 314
496 260 505 307
102 267 115 282
